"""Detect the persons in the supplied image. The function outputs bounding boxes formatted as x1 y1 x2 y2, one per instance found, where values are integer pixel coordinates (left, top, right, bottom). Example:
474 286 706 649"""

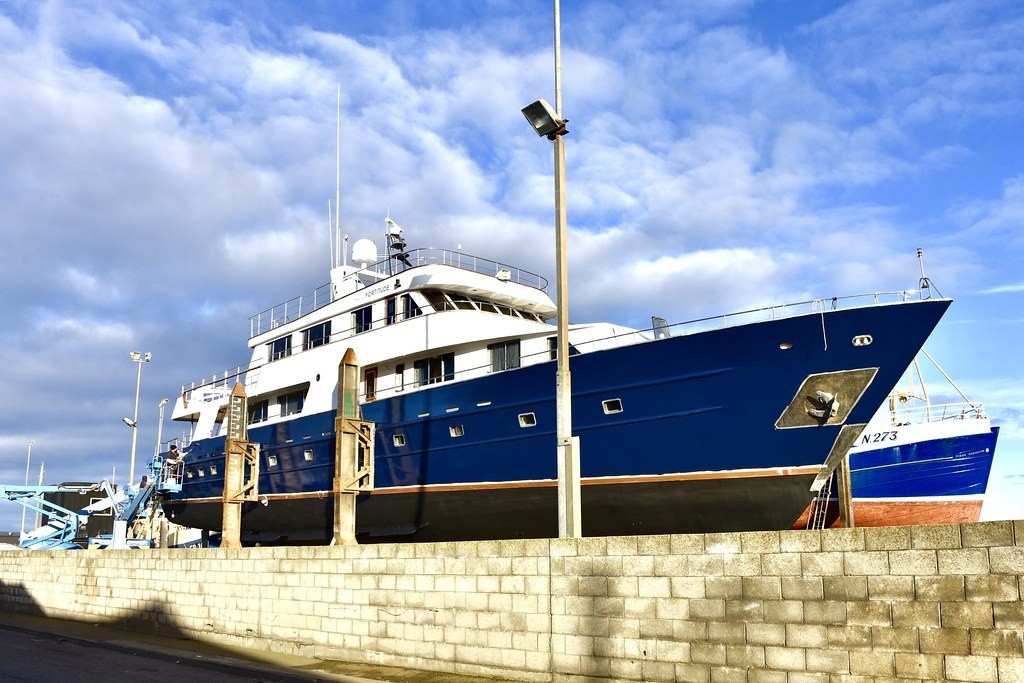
139 476 147 491
165 445 179 477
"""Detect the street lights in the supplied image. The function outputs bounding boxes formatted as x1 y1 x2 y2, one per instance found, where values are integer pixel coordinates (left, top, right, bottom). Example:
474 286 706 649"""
121 351 152 483
21 441 36 531
156 397 170 453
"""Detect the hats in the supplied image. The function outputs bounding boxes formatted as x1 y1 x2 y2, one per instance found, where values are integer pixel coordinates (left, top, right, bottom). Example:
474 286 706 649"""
171 445 177 450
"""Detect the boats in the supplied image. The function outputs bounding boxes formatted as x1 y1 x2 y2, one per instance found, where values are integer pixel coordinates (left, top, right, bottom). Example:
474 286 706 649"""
789 346 1000 531
158 82 958 545
